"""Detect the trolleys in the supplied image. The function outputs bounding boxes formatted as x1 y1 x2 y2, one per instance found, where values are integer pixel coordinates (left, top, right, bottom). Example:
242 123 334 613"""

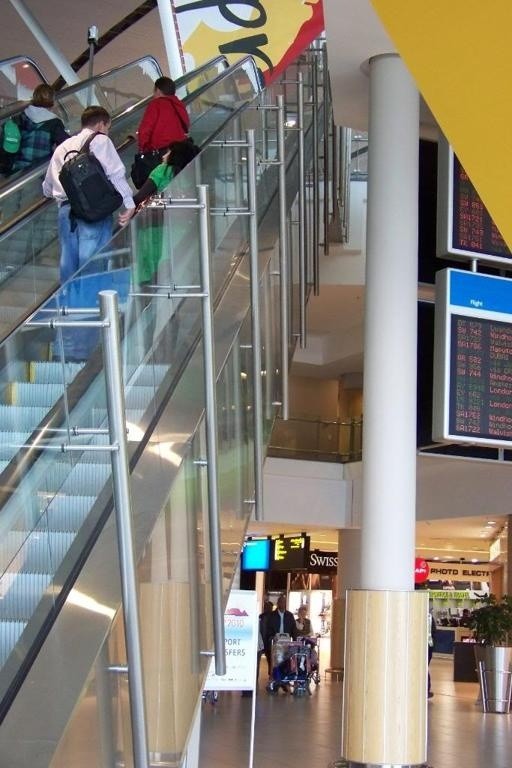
266 633 319 696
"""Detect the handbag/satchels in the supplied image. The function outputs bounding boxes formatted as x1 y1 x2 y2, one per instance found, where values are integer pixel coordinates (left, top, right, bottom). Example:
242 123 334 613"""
59 130 123 224
132 137 201 190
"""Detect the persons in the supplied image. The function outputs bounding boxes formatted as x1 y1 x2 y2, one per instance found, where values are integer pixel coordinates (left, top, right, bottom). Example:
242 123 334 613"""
2 84 71 213
41 106 137 364
460 609 469 627
113 140 202 292
266 597 296 644
259 601 274 676
292 606 313 642
136 76 191 153
428 612 437 699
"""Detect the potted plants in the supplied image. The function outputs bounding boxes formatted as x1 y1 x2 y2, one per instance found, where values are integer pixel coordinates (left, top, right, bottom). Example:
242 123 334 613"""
461 592 512 714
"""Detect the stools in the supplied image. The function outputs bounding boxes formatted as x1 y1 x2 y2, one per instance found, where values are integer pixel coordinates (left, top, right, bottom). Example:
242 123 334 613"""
325 669 343 683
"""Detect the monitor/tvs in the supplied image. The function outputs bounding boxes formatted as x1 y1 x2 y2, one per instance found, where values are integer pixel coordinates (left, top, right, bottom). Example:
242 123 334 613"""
271 532 311 573
241 536 272 571
